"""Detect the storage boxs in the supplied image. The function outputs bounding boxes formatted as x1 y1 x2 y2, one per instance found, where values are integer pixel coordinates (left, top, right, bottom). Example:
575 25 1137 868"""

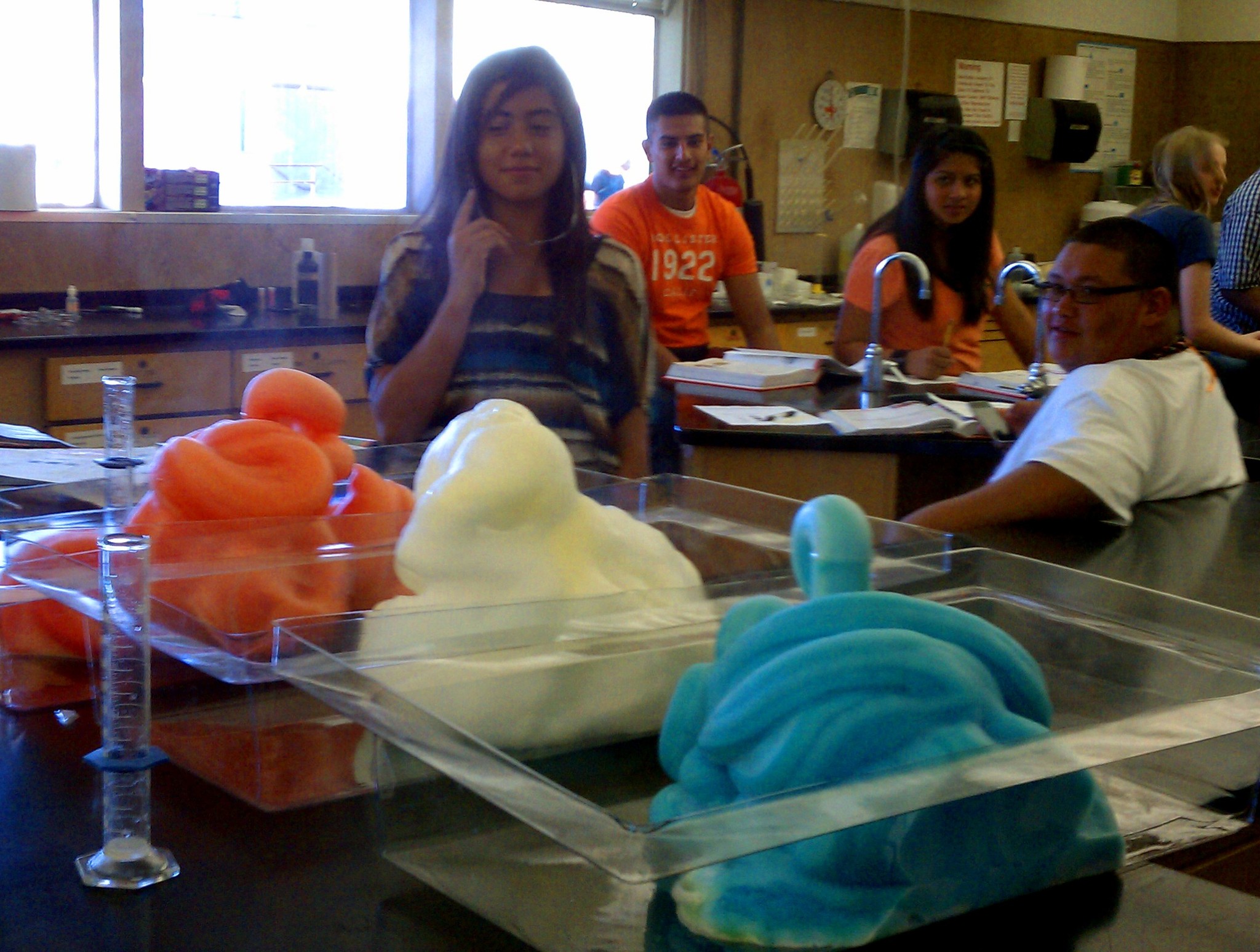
264 541 1260 952
1 472 977 814
1 465 649 714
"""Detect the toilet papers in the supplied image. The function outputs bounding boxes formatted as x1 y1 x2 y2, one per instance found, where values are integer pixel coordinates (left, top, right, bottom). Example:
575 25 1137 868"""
1044 54 1091 100
1 143 39 211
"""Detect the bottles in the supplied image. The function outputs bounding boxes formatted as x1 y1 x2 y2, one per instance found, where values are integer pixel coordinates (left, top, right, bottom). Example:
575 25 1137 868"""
1129 163 1142 186
838 222 866 295
291 237 326 327
65 285 80 322
1007 245 1025 304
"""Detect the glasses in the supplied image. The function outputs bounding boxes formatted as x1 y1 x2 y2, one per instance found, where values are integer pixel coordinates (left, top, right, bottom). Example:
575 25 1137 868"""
1031 280 1158 304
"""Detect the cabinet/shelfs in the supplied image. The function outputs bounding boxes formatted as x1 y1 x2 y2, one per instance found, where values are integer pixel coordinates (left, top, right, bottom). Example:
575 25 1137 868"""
236 342 377 442
41 349 235 456
707 319 748 349
772 317 837 356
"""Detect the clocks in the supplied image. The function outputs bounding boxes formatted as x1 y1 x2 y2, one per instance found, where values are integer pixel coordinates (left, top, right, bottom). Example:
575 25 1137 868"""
813 76 850 131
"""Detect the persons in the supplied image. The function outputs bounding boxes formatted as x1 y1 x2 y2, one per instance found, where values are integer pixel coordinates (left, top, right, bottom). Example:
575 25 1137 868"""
1126 126 1260 415
589 90 781 474
871 215 1250 543
1208 171 1260 360
834 126 1052 382
362 44 650 512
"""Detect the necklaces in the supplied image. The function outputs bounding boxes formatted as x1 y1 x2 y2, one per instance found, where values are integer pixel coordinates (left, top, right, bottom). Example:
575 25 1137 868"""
1138 329 1186 365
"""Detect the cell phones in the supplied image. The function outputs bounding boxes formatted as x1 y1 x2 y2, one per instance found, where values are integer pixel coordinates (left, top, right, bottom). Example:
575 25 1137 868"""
966 399 1020 444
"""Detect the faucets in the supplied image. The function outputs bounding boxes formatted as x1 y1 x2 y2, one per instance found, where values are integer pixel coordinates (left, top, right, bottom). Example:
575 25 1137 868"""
860 250 932 390
994 260 1055 392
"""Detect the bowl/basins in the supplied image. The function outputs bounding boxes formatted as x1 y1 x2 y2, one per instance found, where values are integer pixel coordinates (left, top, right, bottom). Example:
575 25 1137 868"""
710 261 846 307
0 442 1260 952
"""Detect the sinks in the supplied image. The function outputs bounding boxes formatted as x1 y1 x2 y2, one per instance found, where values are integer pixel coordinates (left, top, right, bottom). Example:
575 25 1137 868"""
885 385 1017 412
